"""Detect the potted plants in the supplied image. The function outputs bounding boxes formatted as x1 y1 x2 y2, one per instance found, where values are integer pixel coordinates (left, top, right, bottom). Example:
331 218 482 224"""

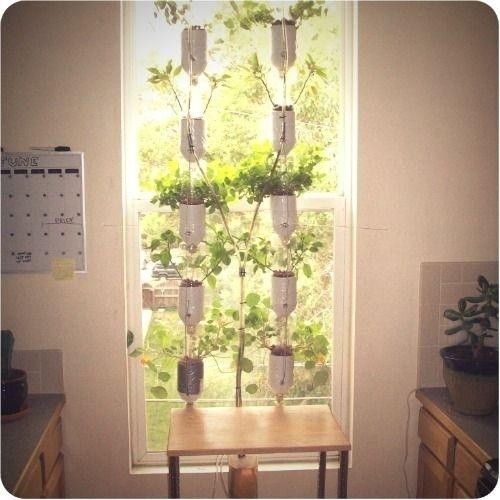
177 97 206 163
163 0 217 83
256 0 328 80
165 173 221 249
442 270 498 417
176 347 208 408
249 254 312 321
269 88 302 159
249 328 330 404
249 162 320 245
174 265 204 337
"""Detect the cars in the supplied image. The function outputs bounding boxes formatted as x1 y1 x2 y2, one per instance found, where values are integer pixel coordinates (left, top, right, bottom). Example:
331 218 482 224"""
151 264 177 279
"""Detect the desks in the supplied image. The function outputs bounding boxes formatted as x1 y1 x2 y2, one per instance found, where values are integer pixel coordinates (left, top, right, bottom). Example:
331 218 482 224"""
3 390 68 499
418 391 495 499
166 406 350 499
